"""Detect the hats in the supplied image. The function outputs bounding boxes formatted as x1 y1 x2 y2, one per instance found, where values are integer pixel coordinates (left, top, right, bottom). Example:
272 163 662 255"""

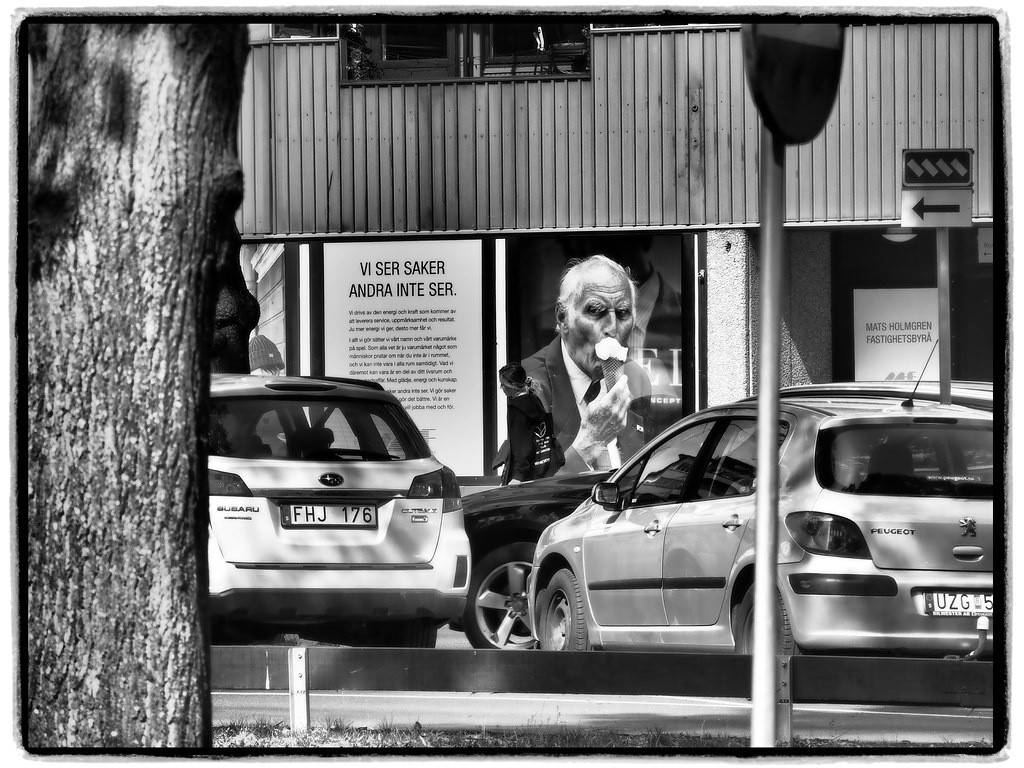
247 334 285 372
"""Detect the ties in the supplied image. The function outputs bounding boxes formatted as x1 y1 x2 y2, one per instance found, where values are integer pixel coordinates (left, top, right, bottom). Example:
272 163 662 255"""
585 380 612 471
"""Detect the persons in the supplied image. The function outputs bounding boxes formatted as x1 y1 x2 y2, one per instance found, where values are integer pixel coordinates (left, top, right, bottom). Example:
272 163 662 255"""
248 334 285 376
491 362 553 486
521 236 682 476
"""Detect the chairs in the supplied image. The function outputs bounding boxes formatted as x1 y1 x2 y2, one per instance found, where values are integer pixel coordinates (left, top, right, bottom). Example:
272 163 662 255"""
868 444 914 482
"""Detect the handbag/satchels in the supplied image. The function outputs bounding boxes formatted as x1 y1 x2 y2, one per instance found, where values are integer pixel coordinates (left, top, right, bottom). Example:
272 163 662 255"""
507 403 553 476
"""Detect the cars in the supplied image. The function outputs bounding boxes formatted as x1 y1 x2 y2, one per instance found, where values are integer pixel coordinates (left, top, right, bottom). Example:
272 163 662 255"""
193 373 473 648
525 393 1000 661
446 379 994 649
